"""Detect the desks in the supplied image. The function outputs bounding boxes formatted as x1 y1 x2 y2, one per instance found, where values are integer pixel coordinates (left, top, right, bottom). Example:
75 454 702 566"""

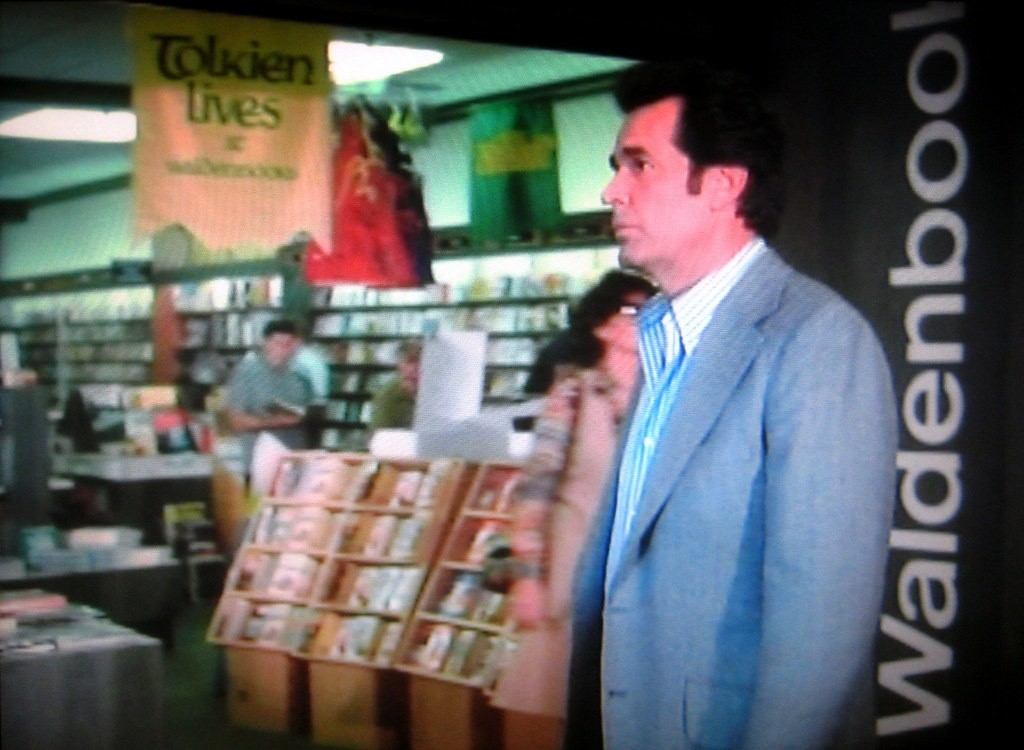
0 588 165 750
0 542 225 656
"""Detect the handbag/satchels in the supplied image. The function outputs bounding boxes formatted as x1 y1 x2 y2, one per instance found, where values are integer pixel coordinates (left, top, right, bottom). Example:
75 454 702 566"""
303 105 439 287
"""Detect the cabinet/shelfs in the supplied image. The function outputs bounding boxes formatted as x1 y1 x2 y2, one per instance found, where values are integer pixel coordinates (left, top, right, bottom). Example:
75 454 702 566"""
0 228 626 455
205 447 538 689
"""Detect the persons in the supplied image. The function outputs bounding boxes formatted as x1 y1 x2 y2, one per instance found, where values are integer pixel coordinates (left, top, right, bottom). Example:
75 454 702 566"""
487 53 897 750
227 319 313 494
173 346 214 412
289 327 330 449
372 341 421 428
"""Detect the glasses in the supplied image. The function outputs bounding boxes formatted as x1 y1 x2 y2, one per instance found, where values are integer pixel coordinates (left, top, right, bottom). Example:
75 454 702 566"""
617 301 641 317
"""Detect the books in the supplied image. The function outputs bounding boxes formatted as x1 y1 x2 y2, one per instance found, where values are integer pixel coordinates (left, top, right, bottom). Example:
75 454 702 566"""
0 261 609 686
